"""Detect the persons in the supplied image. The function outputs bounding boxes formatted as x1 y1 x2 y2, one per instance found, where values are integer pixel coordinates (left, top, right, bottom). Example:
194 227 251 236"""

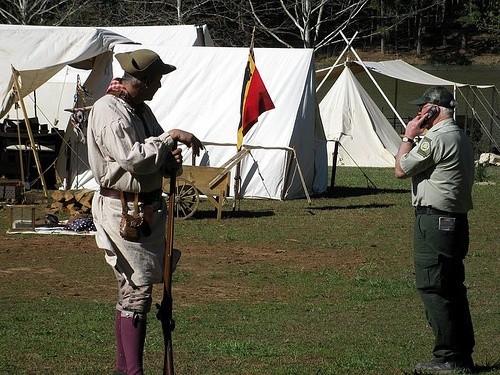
87 50 203 375
395 86 475 371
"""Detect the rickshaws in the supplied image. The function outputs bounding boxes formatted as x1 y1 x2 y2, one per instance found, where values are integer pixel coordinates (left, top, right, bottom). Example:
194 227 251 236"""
161 146 252 222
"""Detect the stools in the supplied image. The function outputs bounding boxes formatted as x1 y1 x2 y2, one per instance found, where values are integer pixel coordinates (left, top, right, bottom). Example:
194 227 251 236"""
0 179 23 205
7 204 39 232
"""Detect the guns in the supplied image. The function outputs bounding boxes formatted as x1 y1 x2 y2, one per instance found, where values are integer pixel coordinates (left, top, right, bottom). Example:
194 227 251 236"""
159 166 175 373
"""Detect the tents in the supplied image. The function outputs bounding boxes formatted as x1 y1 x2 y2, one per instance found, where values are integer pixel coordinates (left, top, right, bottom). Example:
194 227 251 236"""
319 59 499 167
0 25 328 200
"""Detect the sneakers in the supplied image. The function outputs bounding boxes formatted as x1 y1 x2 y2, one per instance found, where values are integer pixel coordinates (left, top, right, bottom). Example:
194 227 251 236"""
416 361 474 373
112 368 128 375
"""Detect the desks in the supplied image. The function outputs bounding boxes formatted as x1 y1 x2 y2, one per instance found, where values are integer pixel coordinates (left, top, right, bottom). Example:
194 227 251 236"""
0 131 72 191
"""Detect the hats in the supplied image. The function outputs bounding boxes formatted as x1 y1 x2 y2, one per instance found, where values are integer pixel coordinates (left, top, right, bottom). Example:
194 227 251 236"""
114 49 176 77
407 86 456 109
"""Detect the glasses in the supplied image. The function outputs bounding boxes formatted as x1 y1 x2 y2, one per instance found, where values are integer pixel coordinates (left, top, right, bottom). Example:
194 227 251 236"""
417 105 433 112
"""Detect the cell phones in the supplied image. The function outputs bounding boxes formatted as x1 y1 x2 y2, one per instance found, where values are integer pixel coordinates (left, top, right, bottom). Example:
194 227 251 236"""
419 106 437 129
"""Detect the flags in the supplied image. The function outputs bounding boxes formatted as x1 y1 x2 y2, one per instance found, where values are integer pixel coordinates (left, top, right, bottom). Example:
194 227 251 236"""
237 46 275 150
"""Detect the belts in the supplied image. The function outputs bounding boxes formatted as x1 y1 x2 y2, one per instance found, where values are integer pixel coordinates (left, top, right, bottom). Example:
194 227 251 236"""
414 205 450 215
99 186 162 203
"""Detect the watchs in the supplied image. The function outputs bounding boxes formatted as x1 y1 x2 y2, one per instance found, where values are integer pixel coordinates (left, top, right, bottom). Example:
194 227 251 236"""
402 137 415 145
